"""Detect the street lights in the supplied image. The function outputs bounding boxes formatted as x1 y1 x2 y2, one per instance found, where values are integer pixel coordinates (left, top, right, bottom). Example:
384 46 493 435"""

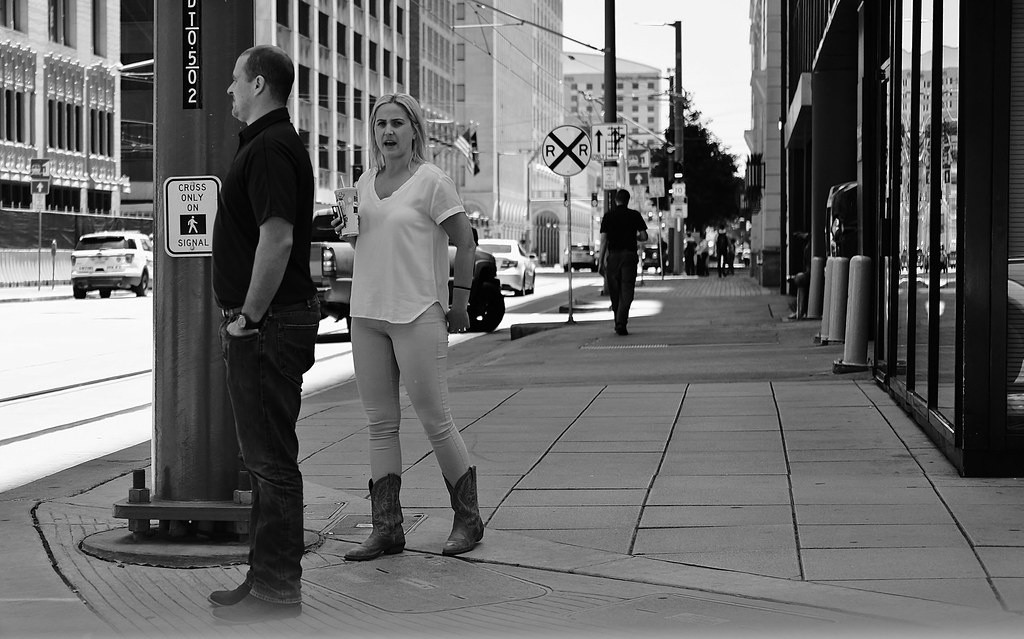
642 20 688 278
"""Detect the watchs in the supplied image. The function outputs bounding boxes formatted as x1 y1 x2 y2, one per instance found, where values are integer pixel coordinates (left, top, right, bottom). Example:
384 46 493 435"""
235 312 260 330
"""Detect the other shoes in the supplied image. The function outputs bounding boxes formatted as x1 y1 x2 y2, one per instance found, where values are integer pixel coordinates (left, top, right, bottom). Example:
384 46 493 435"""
209 590 302 624
209 581 251 607
616 325 628 336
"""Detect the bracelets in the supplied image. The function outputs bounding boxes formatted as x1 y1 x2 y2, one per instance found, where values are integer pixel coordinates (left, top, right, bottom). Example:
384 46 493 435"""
452 285 472 291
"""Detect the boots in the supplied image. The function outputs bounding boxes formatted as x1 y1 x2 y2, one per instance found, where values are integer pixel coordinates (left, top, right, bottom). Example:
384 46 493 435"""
442 465 483 555
344 473 405 560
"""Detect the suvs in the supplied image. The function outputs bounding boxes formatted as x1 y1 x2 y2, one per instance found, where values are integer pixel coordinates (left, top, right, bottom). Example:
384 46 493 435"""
69 228 154 300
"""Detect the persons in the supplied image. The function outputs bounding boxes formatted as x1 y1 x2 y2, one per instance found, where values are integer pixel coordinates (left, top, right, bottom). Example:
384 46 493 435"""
540 249 547 267
657 237 668 275
683 224 752 279
597 188 649 337
210 43 319 626
330 92 485 562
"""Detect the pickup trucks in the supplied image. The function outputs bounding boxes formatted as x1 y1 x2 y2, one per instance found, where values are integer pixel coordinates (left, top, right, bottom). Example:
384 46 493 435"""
309 208 509 332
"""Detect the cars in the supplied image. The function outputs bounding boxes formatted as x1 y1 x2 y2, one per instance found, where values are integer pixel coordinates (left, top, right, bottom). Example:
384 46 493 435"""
563 243 596 272
477 237 537 297
640 244 660 270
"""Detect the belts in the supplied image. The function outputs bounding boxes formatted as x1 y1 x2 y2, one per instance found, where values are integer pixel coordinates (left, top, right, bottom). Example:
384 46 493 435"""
221 307 245 317
